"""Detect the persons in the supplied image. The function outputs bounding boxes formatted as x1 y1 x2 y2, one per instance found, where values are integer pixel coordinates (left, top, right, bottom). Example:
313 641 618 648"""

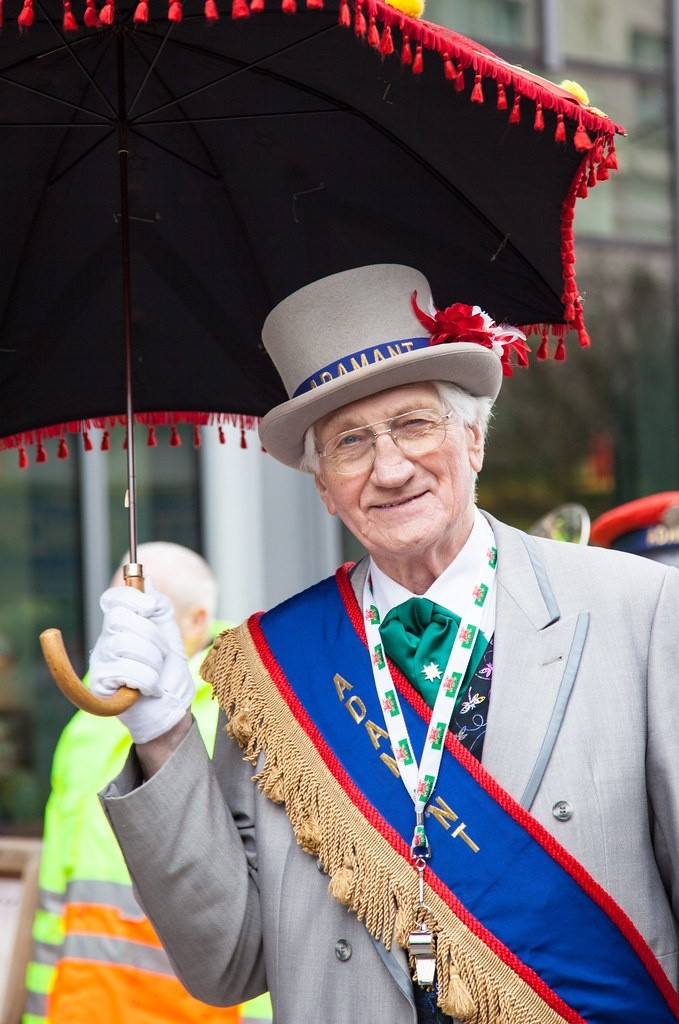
87 264 679 1024
19 541 273 1024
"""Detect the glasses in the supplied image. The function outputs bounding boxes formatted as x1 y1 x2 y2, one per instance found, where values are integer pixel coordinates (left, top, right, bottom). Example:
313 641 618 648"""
313 408 454 476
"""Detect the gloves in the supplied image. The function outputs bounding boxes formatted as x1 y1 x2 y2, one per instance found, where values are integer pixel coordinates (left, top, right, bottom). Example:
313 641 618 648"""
89 581 194 746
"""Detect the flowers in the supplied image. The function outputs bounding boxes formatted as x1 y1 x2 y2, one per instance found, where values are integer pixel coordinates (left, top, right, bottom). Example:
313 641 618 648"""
411 289 532 379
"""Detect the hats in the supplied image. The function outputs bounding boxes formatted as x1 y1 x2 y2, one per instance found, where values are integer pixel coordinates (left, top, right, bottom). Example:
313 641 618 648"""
592 491 679 552
258 263 503 472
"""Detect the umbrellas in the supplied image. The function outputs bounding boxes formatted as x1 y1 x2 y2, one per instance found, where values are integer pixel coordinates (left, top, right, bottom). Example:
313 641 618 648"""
0 0 626 717
